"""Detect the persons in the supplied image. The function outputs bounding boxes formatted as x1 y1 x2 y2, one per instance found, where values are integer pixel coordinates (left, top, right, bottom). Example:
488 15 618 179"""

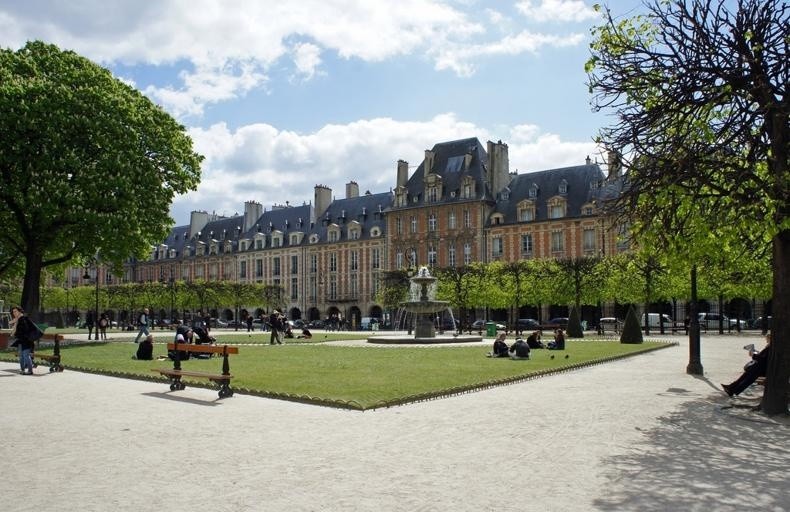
135 335 155 360
547 328 566 350
683 315 691 336
720 329 771 396
536 329 544 347
261 309 313 344
246 314 254 331
527 332 541 349
134 306 149 344
323 313 349 332
98 313 109 340
168 322 215 360
86 310 95 340
191 311 201 329
10 306 44 376
508 336 531 360
202 312 210 331
493 332 510 354
548 328 558 345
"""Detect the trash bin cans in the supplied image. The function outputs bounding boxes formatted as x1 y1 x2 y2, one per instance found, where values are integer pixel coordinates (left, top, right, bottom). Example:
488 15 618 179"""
486 322 496 337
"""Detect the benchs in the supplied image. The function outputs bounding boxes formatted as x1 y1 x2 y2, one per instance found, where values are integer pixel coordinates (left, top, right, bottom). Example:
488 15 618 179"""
12 333 65 373
150 343 239 398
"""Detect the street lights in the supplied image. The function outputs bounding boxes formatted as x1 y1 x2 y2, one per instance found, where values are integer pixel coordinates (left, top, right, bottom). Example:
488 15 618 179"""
404 247 418 279
80 254 99 340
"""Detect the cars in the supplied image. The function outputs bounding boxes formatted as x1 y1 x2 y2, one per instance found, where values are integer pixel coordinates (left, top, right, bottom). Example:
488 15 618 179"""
210 319 324 329
468 318 623 331
361 317 383 330
641 312 772 330
148 319 183 327
